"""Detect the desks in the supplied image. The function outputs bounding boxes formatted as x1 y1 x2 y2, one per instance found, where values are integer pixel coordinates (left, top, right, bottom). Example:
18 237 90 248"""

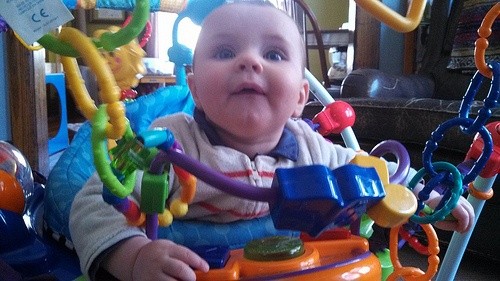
139 73 176 91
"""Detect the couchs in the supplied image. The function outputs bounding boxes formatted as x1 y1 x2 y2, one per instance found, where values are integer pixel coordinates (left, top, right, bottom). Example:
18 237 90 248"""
300 0 500 170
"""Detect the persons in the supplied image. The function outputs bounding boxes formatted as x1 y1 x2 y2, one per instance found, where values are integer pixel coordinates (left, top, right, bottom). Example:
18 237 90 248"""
68 0 476 281
321 22 350 88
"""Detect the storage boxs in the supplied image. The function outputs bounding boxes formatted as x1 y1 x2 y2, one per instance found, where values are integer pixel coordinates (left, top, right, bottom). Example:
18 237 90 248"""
44 72 71 158
